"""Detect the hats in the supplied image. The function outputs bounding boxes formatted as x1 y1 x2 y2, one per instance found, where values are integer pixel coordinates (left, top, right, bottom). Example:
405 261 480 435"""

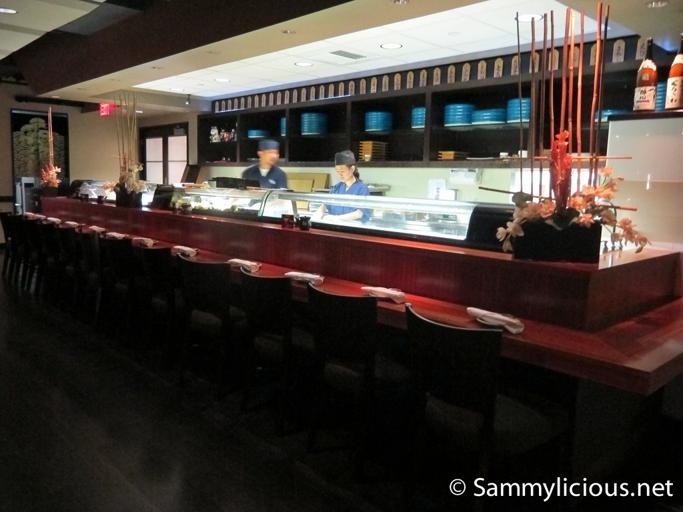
257 140 280 149
334 149 356 164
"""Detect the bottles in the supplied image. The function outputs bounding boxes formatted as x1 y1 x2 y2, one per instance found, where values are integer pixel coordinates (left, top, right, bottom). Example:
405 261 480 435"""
632 33 683 111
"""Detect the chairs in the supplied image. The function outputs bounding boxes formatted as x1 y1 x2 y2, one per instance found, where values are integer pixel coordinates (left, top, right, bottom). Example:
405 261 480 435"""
1 210 548 512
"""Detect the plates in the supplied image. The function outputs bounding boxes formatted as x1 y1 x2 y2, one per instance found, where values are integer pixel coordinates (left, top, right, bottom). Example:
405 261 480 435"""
246 157 258 162
594 109 629 123
247 113 328 137
365 98 531 132
358 141 387 162
436 150 496 160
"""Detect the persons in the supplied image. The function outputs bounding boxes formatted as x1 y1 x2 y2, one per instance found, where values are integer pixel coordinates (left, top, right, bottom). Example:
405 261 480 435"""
308 146 375 229
237 137 289 190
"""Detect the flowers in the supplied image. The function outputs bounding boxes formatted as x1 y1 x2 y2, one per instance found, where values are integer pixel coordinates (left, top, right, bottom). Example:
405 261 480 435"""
41 166 62 187
103 164 147 193
494 168 651 254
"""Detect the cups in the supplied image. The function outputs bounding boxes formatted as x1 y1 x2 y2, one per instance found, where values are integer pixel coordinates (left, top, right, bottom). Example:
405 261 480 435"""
517 150 529 158
281 214 311 231
500 151 508 158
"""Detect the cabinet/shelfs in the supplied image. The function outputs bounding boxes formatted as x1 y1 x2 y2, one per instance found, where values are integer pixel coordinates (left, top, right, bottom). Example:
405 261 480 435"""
196 58 675 164
69 180 513 252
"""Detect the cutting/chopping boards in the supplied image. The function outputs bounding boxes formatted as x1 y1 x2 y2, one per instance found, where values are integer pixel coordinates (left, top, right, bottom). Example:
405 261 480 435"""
284 172 330 210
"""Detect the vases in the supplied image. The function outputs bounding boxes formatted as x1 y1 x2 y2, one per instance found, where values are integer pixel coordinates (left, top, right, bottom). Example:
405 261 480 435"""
512 221 602 265
42 187 58 197
116 193 141 207
